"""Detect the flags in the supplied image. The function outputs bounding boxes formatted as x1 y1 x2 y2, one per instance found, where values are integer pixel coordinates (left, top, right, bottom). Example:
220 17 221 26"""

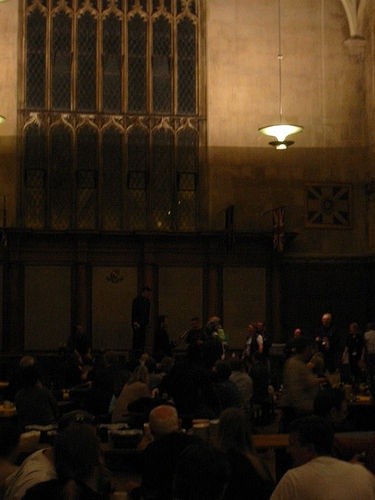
271 207 286 252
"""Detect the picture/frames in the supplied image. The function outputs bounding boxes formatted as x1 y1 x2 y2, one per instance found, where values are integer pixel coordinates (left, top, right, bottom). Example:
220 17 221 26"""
304 182 354 230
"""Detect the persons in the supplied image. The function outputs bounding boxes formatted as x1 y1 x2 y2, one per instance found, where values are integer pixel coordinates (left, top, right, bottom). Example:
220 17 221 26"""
0 288 375 500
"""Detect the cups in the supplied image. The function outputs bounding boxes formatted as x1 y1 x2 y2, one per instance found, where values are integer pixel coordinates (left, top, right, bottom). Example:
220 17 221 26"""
4 401 11 408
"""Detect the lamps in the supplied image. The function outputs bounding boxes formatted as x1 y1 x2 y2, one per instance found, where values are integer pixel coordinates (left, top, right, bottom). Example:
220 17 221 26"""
257 0 304 151
